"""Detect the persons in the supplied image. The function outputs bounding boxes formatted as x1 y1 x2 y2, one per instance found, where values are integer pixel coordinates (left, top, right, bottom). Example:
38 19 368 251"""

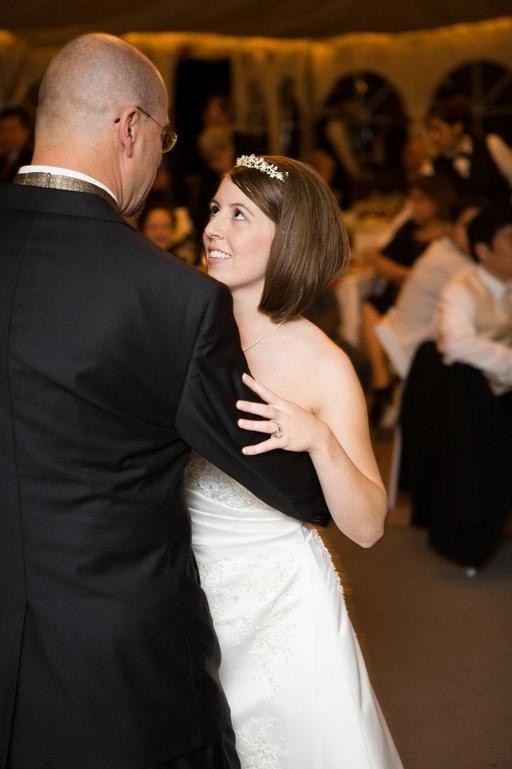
3 29 512 568
179 153 406 769
0 33 336 769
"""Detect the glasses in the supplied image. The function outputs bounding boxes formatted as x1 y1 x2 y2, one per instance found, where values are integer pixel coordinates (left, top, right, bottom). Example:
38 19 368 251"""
116 105 178 152
